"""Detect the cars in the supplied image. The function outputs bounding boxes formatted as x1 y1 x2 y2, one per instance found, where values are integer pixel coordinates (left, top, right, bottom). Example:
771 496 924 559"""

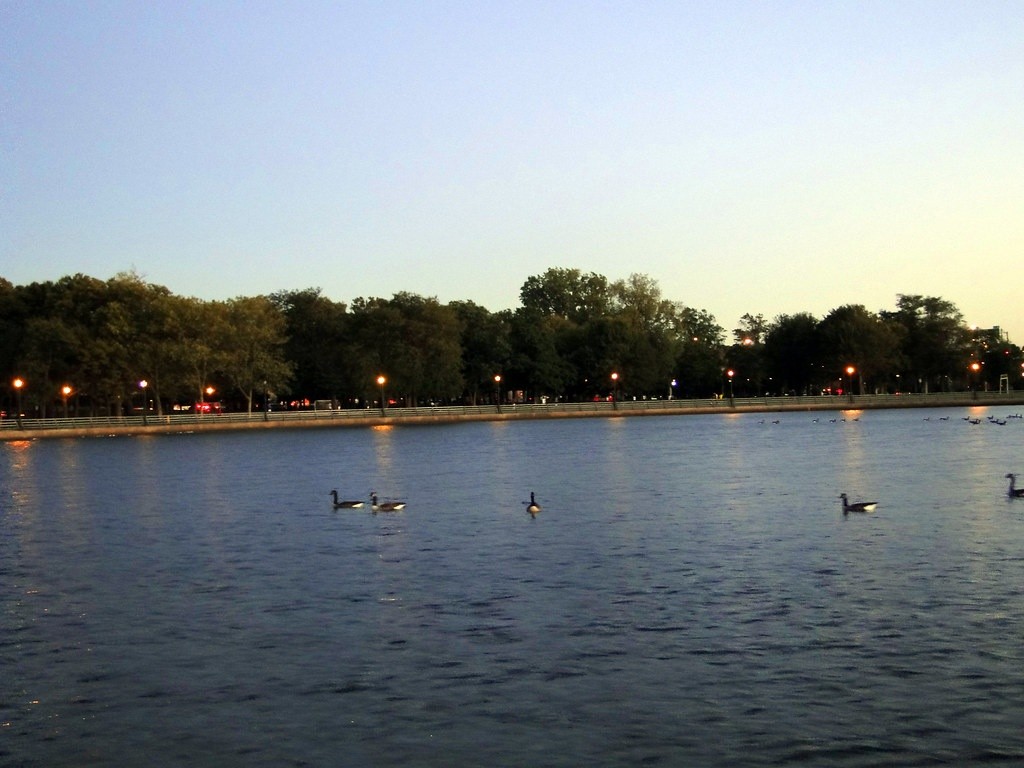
188 401 222 414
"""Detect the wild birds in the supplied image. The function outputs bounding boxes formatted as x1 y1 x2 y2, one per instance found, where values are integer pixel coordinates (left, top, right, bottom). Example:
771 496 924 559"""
369 492 406 510
1005 473 1024 497
328 490 366 508
839 494 877 512
527 492 541 512
757 414 1022 425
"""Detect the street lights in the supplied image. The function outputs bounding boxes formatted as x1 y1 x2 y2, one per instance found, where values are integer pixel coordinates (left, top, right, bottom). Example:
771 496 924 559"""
612 373 618 410
14 378 26 430
139 380 149 426
60 385 71 417
495 375 503 414
846 367 855 404
728 370 736 408
972 363 980 401
377 375 387 418
